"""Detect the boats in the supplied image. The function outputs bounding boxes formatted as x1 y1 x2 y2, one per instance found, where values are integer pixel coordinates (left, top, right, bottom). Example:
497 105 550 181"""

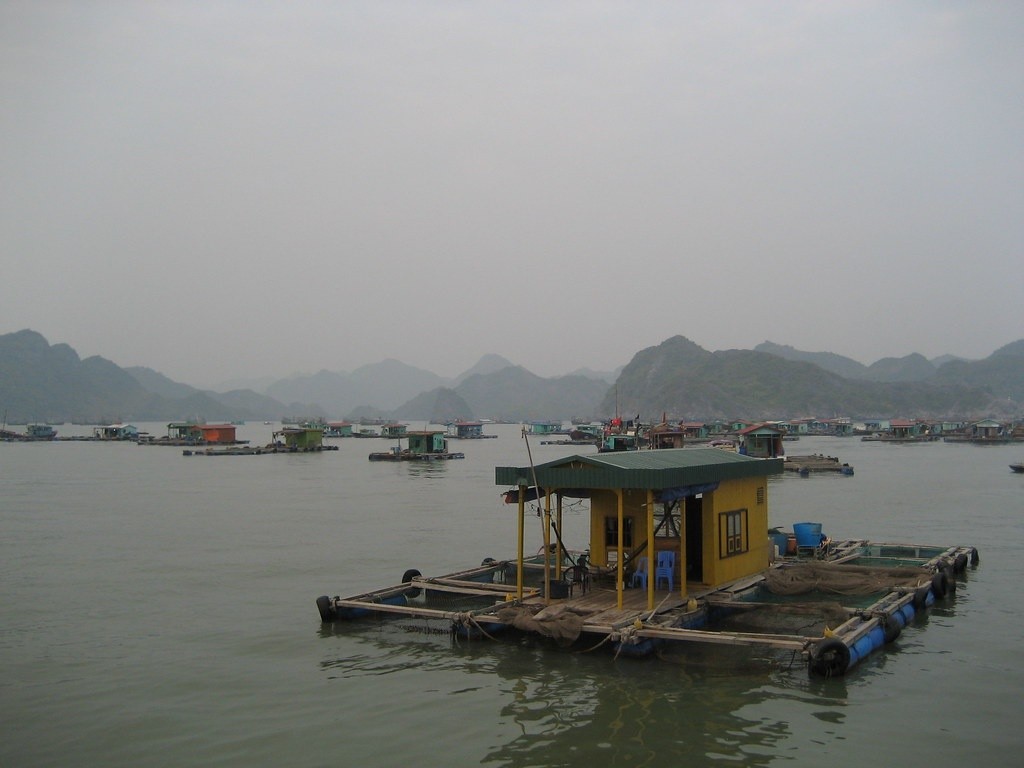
478 418 496 424
360 416 398 425
523 420 572 434
368 431 465 462
14 424 58 442
0 428 23 441
137 423 208 445
736 423 850 476
350 424 448 439
566 412 1024 447
178 424 250 446
224 419 246 426
281 417 327 425
55 424 149 441
182 428 339 456
444 422 499 439
1008 463 1024 472
314 447 980 679
297 422 354 438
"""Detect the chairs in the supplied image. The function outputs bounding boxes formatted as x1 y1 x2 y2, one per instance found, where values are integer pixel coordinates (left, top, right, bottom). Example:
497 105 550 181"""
655 550 675 592
563 566 591 598
631 556 649 593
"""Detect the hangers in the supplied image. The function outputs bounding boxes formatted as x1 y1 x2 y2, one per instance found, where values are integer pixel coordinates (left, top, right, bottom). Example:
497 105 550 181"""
524 499 590 521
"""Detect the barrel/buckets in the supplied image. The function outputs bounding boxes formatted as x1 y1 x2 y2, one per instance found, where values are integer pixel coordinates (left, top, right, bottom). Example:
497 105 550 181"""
792 522 822 555
766 532 788 554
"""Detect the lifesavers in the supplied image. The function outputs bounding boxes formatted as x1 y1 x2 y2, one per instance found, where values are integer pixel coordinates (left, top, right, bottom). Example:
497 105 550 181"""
255 446 339 455
934 572 947 595
479 557 495 577
402 569 421 596
424 454 430 461
809 638 850 676
956 554 969 575
